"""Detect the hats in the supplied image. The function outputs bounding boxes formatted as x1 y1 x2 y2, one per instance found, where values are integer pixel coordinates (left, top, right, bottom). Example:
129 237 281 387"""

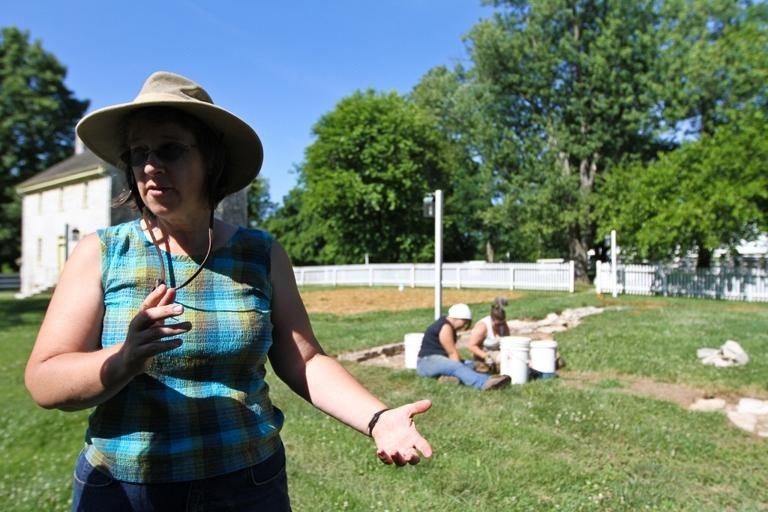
69 70 265 212
448 303 473 323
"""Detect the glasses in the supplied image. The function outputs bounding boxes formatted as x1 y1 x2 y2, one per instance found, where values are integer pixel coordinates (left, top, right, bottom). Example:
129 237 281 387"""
117 138 203 169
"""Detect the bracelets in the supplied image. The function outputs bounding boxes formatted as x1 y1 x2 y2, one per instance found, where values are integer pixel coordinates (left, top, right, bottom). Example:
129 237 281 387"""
368 408 390 438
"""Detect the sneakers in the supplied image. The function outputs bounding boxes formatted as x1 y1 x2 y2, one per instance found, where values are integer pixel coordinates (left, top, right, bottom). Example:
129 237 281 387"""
483 373 512 392
438 375 460 387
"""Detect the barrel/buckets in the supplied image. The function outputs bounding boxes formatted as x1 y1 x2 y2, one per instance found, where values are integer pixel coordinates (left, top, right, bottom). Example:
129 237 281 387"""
500 335 530 386
404 332 425 369
529 339 558 379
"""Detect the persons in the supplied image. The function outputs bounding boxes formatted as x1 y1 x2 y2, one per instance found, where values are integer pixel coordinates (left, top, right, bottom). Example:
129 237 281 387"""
25 73 434 512
467 305 510 374
417 304 510 394
